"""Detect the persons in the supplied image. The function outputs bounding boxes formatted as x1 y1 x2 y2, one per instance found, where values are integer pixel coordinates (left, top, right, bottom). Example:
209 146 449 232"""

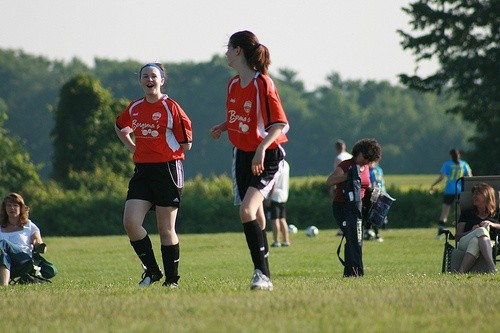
0 192 46 286
429 147 473 239
209 30 290 291
456 183 500 274
325 138 385 278
115 63 193 289
270 159 291 247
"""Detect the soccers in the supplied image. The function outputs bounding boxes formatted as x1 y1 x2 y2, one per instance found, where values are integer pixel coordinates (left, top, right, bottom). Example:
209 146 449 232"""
286 224 299 235
305 224 320 238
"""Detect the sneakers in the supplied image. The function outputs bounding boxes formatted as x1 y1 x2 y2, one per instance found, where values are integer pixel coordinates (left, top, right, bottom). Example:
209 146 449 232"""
162 280 177 290
272 242 281 247
283 241 289 246
138 271 164 286
250 270 274 291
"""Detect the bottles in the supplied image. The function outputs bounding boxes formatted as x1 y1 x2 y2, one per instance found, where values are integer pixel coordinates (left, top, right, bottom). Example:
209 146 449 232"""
370 183 381 203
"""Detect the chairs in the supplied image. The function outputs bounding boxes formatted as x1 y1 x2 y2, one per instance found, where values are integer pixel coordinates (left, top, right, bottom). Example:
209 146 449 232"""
438 171 500 273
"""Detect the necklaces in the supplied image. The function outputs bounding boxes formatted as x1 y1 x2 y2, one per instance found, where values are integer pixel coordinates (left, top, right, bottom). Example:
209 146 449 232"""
7 221 19 226
